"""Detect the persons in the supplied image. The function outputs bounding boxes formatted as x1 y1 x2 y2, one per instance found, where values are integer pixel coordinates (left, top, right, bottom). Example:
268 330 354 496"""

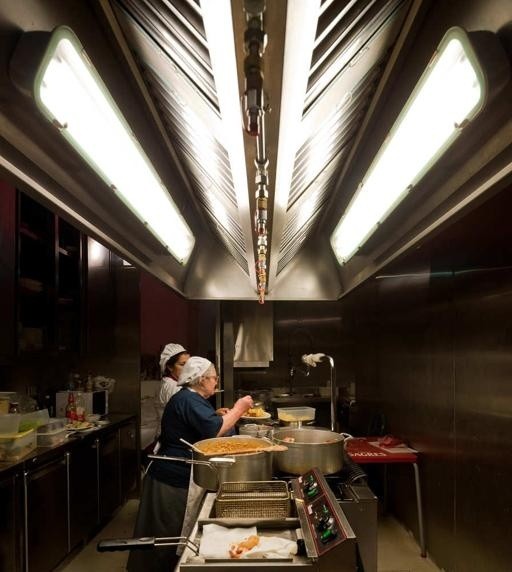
159 343 229 433
123 357 254 570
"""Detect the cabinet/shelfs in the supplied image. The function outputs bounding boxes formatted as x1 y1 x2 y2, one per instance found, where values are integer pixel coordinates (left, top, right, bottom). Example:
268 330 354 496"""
1 422 138 572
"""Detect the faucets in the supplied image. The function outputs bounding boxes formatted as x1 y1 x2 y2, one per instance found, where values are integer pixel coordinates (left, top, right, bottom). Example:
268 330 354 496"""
287 326 317 395
305 354 336 433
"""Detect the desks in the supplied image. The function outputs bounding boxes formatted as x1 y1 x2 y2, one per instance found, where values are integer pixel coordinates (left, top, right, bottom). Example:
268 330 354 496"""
344 435 427 557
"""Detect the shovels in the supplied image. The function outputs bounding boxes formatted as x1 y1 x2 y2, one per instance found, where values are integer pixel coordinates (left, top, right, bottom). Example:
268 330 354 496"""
203 444 289 457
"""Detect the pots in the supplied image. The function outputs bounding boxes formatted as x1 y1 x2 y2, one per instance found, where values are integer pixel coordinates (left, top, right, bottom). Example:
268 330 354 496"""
273 428 345 476
191 435 275 492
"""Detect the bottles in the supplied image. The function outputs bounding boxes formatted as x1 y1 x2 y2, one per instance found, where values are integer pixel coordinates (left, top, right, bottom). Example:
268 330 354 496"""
65 390 78 421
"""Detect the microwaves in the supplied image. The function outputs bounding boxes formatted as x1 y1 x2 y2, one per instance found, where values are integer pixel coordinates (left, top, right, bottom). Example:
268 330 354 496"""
56 387 110 419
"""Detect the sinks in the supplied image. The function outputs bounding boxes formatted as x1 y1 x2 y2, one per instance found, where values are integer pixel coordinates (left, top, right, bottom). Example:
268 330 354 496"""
272 394 318 401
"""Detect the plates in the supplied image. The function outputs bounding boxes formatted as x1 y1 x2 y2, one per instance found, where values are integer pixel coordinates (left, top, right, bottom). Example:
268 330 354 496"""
227 410 271 419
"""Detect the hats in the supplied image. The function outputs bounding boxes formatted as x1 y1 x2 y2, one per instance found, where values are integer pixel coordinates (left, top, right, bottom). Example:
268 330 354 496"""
175 354 211 387
159 342 187 378
